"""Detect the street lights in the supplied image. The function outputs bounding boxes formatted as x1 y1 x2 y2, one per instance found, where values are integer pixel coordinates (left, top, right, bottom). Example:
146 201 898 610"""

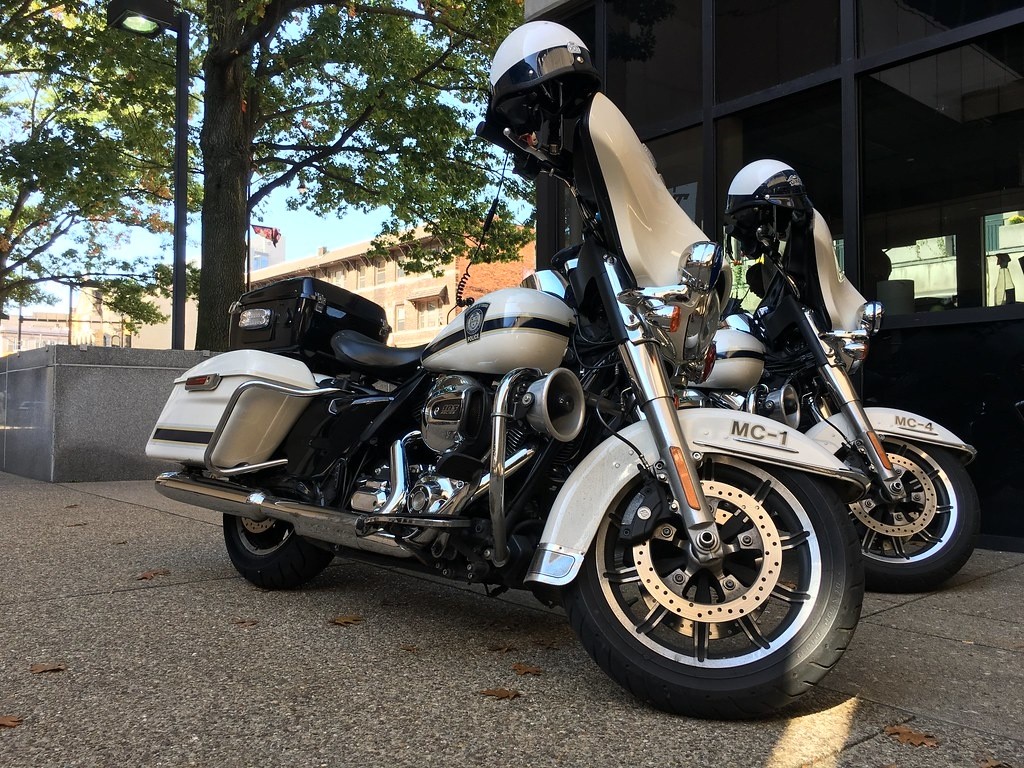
107 1 186 351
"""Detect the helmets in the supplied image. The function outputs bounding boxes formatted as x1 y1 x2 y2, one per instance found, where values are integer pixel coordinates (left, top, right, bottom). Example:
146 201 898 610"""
725 158 813 214
488 20 603 113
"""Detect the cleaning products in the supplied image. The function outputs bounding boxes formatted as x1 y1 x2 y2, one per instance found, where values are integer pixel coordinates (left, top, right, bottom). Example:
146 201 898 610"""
994 252 1015 306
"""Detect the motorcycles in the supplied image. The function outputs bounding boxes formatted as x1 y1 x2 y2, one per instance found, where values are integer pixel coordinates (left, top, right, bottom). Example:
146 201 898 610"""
144 20 981 724
